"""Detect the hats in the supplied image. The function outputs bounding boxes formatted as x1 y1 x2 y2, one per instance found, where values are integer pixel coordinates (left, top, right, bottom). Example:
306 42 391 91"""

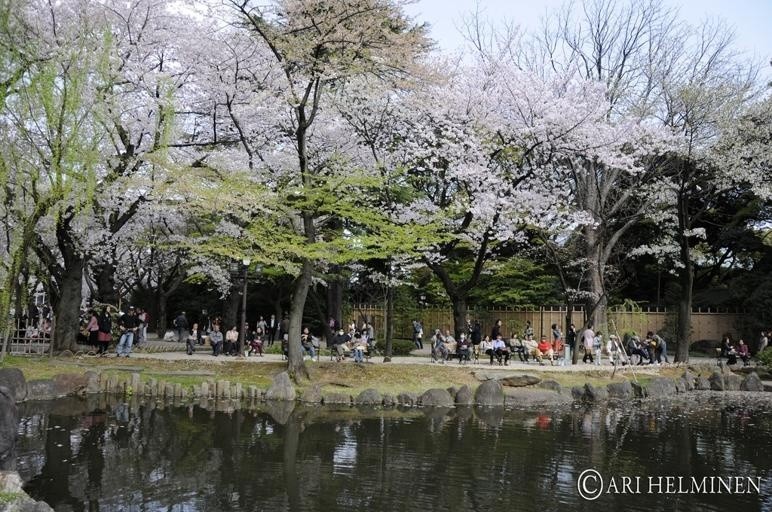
610 335 617 339
129 305 134 309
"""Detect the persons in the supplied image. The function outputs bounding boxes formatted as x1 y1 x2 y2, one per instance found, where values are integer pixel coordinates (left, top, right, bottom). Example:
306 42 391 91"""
411 319 421 349
417 322 424 349
25 306 376 363
721 337 738 366
736 340 750 365
756 330 772 365
430 318 668 365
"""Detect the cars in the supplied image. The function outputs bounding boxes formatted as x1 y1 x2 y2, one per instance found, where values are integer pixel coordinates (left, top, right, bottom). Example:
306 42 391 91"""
690 340 751 360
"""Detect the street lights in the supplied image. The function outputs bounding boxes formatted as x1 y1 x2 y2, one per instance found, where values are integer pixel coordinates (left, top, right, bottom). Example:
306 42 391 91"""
239 257 252 357
